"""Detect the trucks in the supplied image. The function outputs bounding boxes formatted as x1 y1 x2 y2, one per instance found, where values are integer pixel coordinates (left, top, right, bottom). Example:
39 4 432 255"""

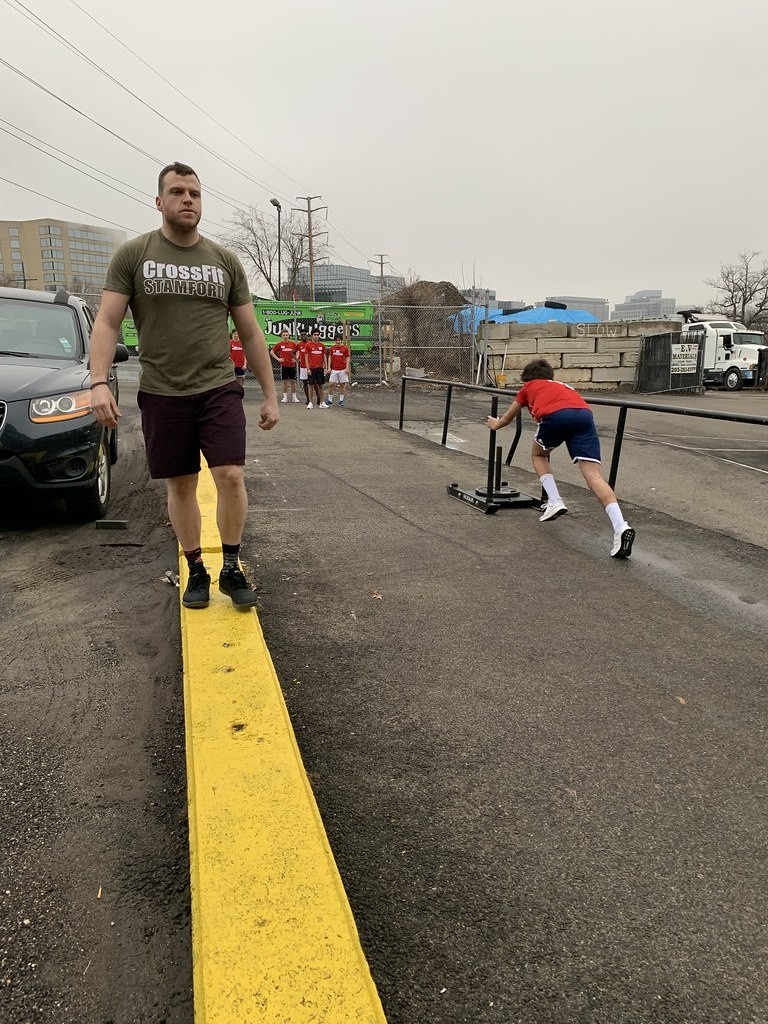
681 314 768 391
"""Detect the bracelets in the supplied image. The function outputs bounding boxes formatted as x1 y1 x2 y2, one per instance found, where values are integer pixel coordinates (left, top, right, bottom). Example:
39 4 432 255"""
89 382 109 391
307 368 309 370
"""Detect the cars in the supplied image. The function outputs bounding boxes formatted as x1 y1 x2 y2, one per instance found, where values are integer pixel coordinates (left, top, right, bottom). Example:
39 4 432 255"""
1 285 131 520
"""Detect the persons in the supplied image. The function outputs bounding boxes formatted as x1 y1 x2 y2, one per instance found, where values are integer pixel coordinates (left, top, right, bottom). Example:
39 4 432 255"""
229 329 248 387
486 359 635 558
324 333 350 406
89 161 280 611
269 330 300 403
291 329 321 405
305 328 330 410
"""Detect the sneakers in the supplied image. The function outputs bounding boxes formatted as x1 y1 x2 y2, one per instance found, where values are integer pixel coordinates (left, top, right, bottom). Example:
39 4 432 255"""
217 570 258 608
319 402 329 409
609 522 635 560
307 403 314 410
182 571 211 609
539 496 567 521
305 401 310 407
326 400 333 407
291 398 299 402
339 401 344 407
281 398 288 402
317 400 320 405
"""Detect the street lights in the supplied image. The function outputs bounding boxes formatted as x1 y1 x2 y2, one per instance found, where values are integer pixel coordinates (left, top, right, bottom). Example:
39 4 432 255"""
269 197 283 301
